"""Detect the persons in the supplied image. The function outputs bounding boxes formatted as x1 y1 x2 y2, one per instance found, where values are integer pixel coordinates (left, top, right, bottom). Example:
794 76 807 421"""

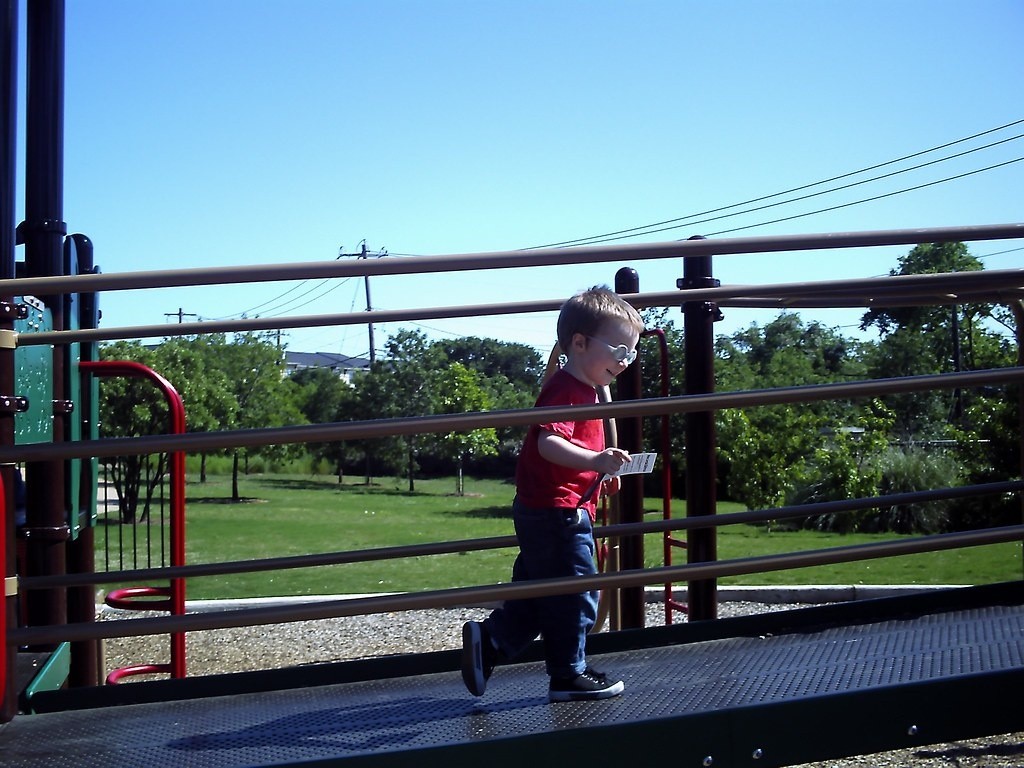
462 286 644 701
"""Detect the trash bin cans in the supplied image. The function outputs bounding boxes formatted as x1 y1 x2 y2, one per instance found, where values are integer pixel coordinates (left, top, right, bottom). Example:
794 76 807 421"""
12 474 48 652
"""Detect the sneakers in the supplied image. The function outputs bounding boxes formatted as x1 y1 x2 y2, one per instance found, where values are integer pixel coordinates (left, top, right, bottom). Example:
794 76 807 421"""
461 621 496 697
550 664 624 700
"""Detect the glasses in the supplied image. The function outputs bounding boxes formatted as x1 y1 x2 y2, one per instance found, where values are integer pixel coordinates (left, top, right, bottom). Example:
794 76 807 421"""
587 336 638 365
